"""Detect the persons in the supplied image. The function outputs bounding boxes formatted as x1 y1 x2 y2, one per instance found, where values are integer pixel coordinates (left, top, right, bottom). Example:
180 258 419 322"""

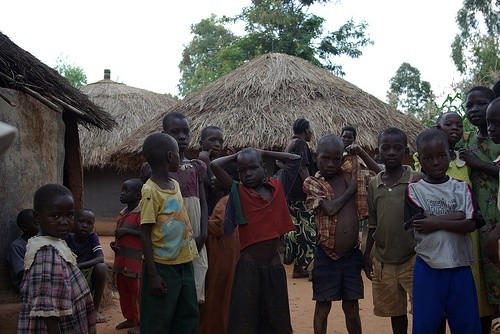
160 113 209 304
110 178 144 334
363 127 426 334
199 161 239 334
6 209 40 291
413 110 492 334
281 117 319 279
196 126 237 216
139 133 201 334
303 133 383 334
211 147 303 334
62 209 107 324
16 183 97 334
404 128 486 334
458 80 500 334
341 125 358 146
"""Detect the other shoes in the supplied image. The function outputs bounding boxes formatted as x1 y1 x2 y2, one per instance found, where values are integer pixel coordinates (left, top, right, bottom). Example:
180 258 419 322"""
292 265 309 278
116 321 136 329
127 326 141 334
